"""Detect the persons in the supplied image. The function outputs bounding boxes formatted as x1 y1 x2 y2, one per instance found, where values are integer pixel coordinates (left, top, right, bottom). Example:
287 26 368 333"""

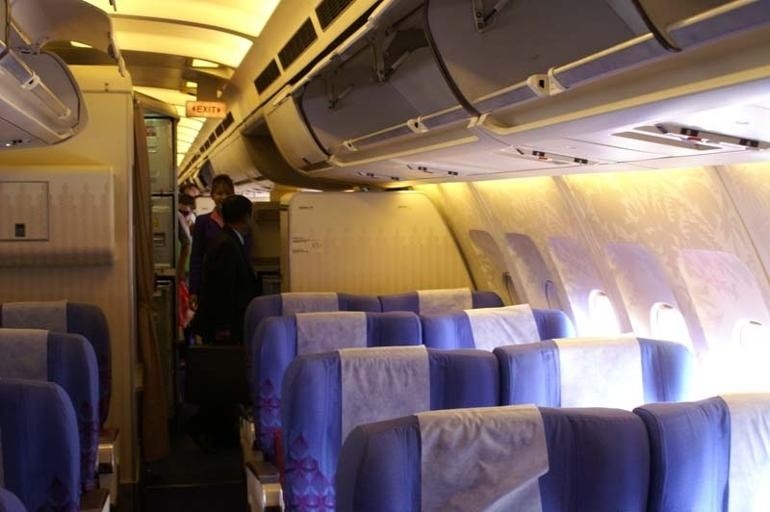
170 173 263 435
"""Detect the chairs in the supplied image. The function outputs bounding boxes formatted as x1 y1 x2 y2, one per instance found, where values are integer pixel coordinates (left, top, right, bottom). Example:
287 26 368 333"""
238 287 768 511
0 299 122 511
